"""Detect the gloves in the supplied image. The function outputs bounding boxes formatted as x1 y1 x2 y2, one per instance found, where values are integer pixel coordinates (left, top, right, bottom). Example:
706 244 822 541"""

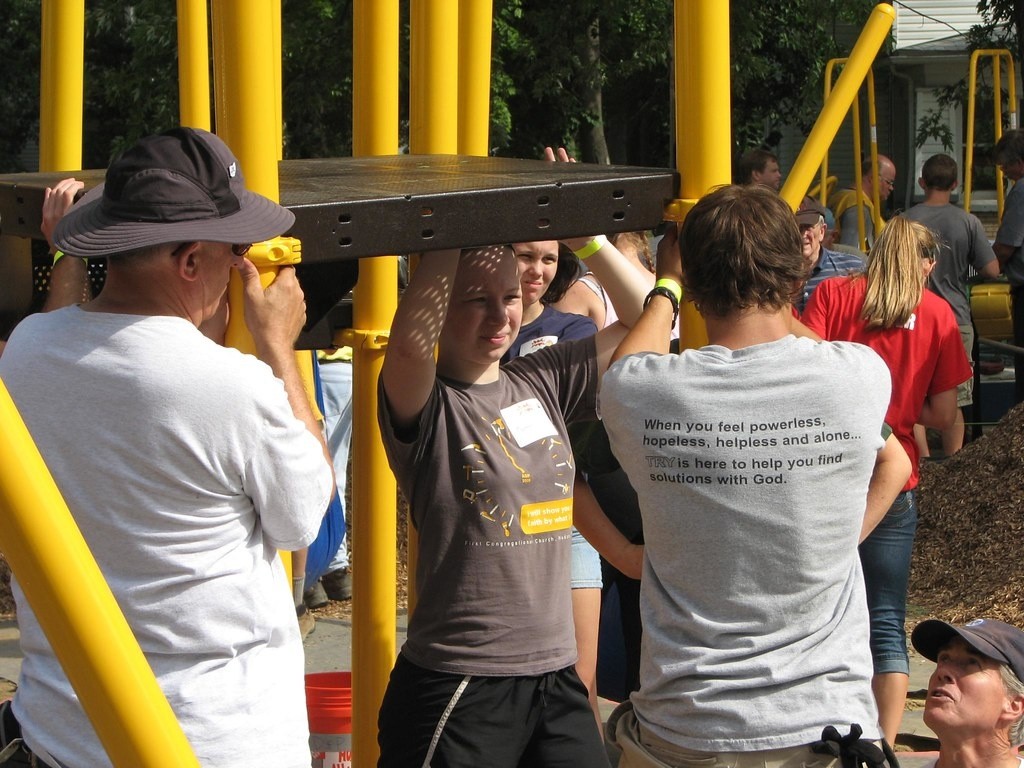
811 723 899 768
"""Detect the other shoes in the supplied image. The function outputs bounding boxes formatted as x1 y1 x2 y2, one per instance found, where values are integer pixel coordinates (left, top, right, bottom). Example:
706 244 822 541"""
325 568 354 600
293 601 316 642
305 579 329 607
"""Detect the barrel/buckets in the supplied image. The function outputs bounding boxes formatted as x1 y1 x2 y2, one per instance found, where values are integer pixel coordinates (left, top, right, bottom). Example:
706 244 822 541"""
305 671 352 768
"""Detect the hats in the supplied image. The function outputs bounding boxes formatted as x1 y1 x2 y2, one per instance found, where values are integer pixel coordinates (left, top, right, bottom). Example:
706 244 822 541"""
52 127 295 257
911 618 1024 683
795 195 826 225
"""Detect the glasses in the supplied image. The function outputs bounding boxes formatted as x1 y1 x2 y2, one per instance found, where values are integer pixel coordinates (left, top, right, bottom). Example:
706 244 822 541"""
879 173 897 187
172 240 250 255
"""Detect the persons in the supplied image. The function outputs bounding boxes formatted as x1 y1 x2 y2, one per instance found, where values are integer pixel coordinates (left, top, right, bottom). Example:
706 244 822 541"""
912 619 1024 768
991 130 1024 404
828 154 896 248
859 425 912 544
0 129 354 767
757 131 782 155
822 209 867 264
599 186 892 767
499 239 603 743
376 147 681 768
788 217 973 755
900 154 1000 457
738 151 781 190
797 194 867 315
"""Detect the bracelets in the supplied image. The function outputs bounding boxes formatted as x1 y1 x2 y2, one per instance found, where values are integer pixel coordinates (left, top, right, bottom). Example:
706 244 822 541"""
642 287 679 330
52 250 88 269
655 279 681 303
574 235 606 259
